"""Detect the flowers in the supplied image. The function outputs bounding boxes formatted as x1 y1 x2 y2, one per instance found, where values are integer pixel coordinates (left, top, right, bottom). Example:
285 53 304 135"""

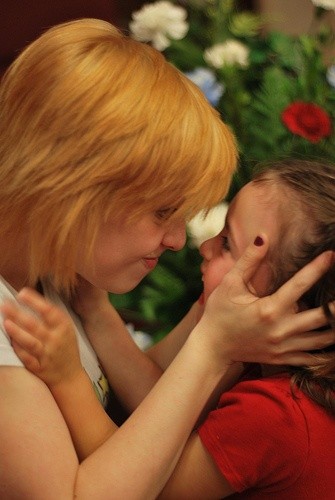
110 0 335 352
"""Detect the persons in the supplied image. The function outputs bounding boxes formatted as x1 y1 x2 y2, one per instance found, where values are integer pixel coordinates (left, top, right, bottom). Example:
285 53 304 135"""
0 17 334 499
2 161 334 500
